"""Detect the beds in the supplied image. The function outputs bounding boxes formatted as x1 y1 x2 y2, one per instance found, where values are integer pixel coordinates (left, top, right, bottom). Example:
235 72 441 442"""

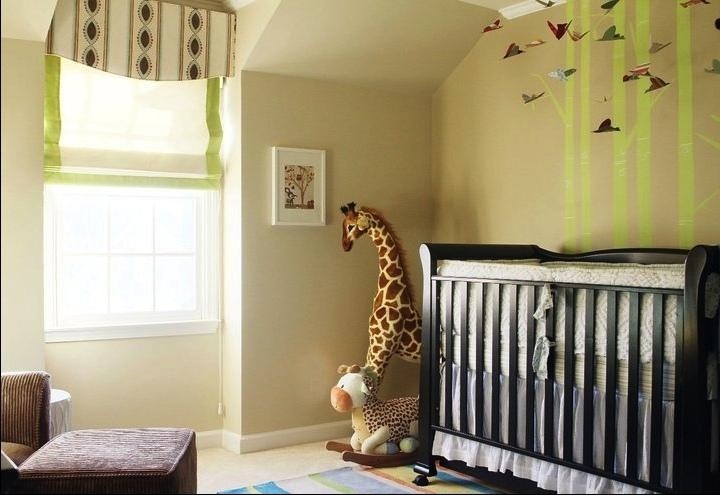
413 241 719 495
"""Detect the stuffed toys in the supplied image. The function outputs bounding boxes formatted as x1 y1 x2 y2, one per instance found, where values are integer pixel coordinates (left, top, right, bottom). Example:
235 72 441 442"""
339 201 423 391
330 365 419 455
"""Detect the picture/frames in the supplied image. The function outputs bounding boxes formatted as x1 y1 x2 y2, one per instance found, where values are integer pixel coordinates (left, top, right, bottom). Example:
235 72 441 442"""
271 146 326 225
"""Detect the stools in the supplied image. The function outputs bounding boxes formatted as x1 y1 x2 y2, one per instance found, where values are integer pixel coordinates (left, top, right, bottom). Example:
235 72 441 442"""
15 428 197 495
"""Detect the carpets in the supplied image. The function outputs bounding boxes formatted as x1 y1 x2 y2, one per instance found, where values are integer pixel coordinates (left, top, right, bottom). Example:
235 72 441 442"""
210 454 513 493
